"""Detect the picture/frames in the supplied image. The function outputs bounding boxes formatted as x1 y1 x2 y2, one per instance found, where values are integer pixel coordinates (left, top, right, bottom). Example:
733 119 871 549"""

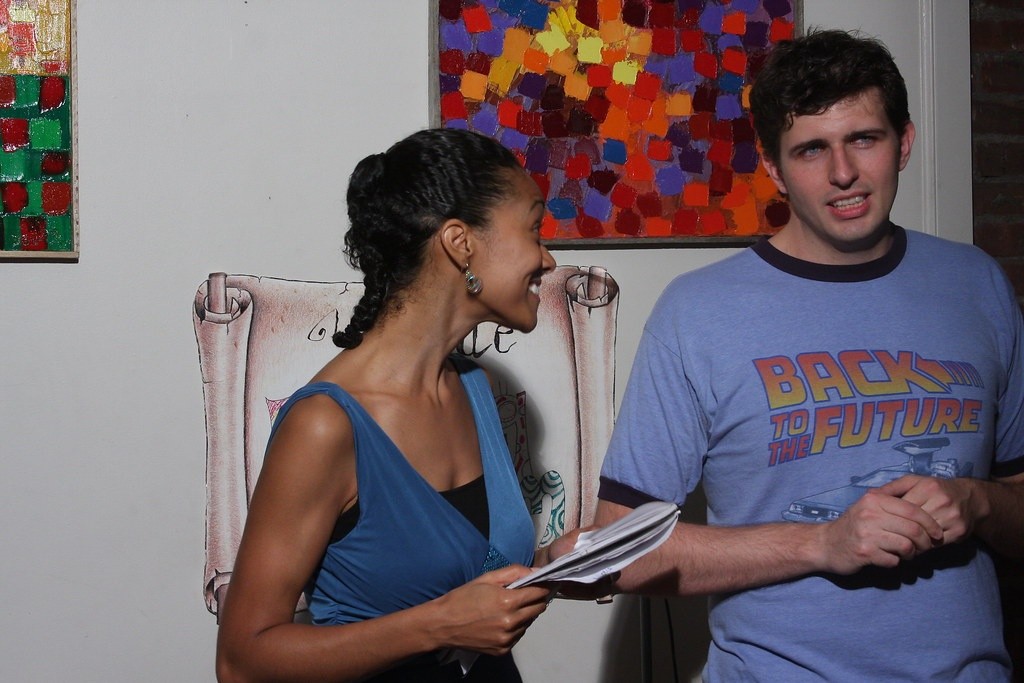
429 0 807 251
0 0 82 262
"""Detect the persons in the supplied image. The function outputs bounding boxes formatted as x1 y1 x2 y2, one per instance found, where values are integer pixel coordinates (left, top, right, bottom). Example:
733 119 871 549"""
216 127 623 683
596 30 1024 683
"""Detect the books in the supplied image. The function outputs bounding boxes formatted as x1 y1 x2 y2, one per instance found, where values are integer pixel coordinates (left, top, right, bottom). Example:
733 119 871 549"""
505 501 682 589
